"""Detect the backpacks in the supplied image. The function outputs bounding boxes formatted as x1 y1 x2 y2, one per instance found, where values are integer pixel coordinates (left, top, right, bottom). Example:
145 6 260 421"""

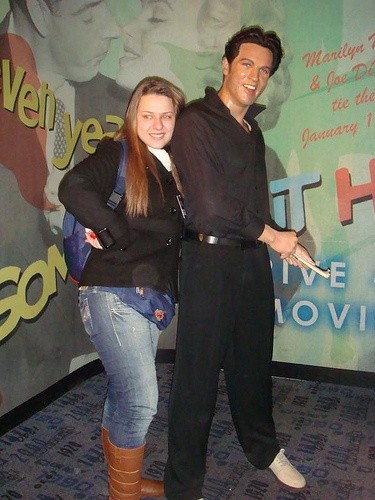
60 136 130 284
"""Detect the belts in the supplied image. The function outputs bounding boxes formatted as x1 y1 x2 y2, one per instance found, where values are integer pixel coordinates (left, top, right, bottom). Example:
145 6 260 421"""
184 231 266 248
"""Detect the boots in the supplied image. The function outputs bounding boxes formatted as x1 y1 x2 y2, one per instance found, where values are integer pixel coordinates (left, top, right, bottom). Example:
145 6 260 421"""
104 437 146 499
101 424 164 497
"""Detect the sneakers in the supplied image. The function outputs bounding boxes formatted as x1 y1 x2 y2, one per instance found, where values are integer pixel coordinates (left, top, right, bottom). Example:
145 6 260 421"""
269 446 309 494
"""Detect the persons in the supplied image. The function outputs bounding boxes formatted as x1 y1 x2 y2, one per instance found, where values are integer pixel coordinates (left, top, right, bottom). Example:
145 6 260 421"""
114 0 319 351
162 24 317 500
57 75 191 500
0 0 136 417
